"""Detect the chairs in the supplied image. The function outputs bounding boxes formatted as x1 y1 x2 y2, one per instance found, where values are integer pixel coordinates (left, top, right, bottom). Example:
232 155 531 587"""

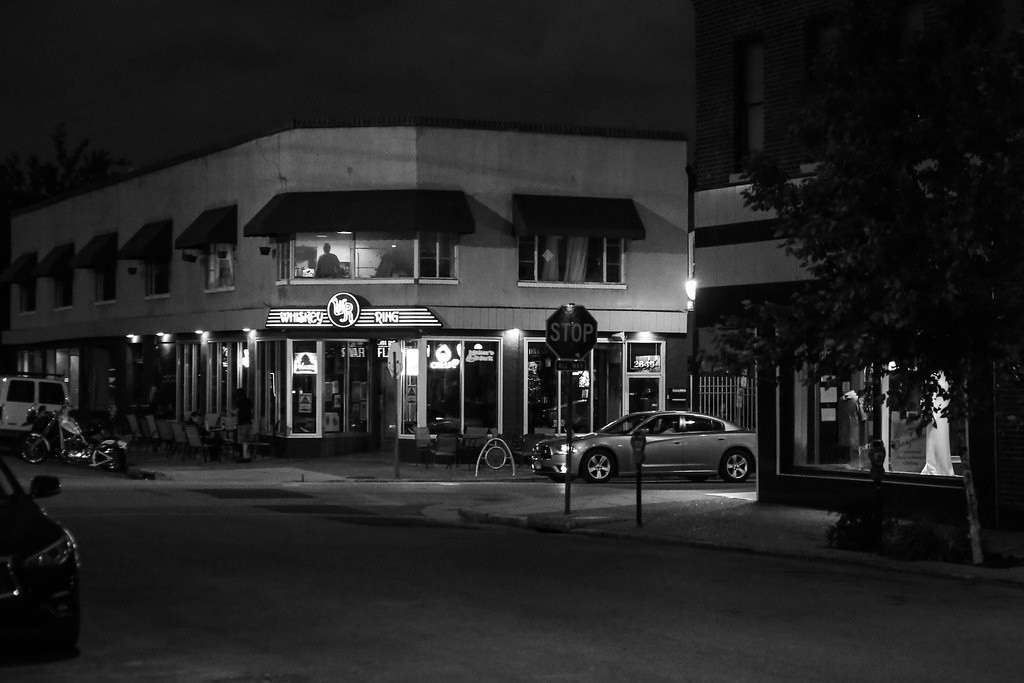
427 427 556 466
127 414 281 464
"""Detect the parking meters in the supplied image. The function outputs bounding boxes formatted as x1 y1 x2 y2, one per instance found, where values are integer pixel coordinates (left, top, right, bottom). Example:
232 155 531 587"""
630 429 647 527
867 438 886 548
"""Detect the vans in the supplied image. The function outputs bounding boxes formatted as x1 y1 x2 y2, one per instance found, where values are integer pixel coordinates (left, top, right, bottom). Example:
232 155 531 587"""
0 371 70 443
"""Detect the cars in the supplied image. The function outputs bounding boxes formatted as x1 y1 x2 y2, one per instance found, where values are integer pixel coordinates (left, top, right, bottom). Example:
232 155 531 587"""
530 410 757 485
0 454 82 653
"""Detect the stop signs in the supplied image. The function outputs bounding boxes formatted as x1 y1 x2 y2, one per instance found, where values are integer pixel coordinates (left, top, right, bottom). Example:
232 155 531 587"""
545 305 597 361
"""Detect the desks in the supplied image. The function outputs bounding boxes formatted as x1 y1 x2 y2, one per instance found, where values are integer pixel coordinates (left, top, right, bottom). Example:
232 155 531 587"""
208 424 243 459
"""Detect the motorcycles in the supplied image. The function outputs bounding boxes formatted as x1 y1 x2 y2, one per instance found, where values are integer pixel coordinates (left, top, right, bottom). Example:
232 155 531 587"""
18 394 129 473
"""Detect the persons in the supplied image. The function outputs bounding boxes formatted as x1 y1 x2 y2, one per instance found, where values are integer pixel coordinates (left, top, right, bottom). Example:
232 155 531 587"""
224 388 253 465
185 413 221 460
